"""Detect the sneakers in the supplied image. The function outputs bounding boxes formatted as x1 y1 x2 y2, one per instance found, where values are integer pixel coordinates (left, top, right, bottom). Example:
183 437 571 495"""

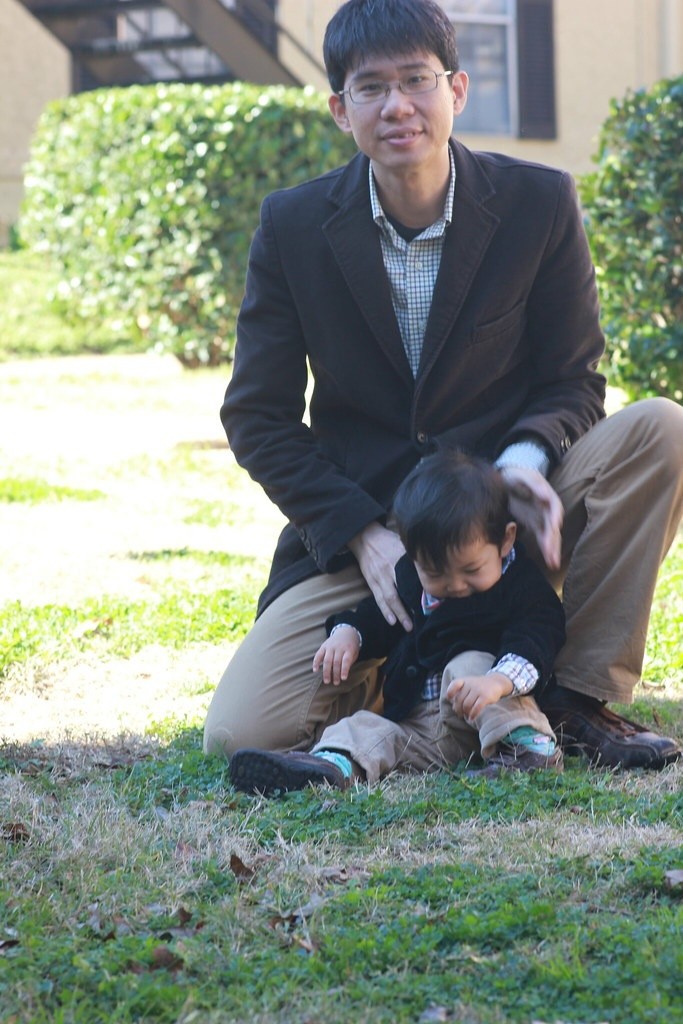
541 693 681 771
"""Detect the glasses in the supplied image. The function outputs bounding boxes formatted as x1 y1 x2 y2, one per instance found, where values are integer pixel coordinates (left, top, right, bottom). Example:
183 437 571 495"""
338 69 454 104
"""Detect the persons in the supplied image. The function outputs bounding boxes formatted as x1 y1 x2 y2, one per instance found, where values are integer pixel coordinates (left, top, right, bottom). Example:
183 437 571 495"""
230 447 565 799
201 0 683 773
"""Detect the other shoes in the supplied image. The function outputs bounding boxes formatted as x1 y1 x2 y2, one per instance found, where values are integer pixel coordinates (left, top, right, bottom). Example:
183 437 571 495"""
230 748 350 799
477 744 565 780
